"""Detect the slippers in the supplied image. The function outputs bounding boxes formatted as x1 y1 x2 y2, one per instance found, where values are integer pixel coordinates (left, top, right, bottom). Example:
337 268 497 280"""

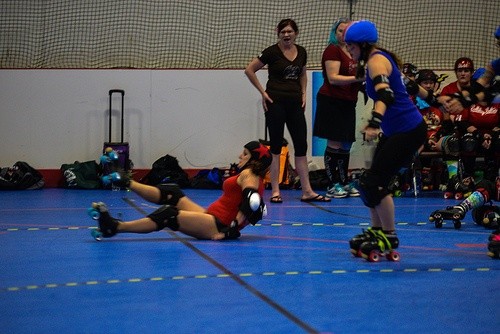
301 194 331 202
270 196 282 203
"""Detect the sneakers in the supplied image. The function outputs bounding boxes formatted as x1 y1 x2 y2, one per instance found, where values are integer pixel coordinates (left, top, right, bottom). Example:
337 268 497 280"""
344 182 361 196
326 183 348 198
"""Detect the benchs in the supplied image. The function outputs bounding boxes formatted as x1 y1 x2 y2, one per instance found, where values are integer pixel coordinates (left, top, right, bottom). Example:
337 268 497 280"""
410 151 485 197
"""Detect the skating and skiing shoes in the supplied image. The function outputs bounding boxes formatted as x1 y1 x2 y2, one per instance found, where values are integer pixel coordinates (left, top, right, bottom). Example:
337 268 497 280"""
475 205 500 229
439 174 472 199
429 206 465 228
421 168 434 190
100 147 131 187
87 202 121 241
350 227 400 262
387 173 410 196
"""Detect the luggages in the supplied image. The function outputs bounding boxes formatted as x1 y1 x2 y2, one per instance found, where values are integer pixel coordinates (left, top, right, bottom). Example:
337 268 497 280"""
103 89 130 177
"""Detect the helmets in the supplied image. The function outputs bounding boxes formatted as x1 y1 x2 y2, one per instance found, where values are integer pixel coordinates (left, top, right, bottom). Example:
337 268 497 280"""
342 20 378 43
402 63 417 75
235 141 273 170
455 58 474 73
415 69 437 83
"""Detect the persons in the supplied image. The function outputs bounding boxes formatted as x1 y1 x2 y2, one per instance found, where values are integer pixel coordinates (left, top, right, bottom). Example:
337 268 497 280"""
87 141 273 242
389 26 500 260
245 19 331 203
342 21 448 263
313 17 366 199
